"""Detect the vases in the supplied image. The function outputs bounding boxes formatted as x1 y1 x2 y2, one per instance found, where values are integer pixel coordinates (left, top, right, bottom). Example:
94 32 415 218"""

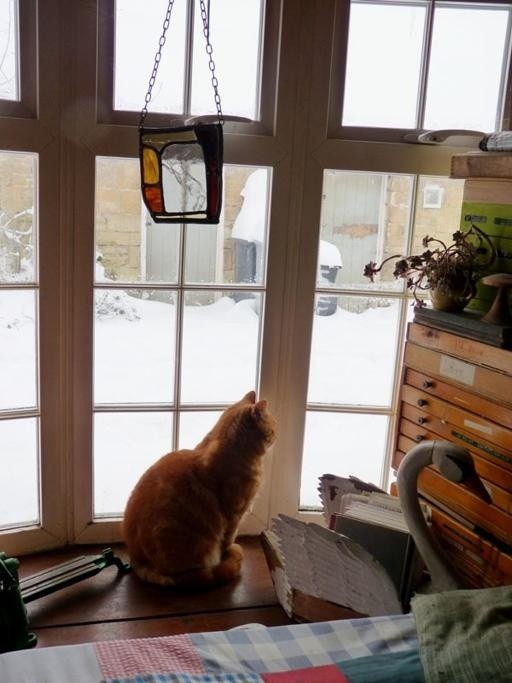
430 288 464 312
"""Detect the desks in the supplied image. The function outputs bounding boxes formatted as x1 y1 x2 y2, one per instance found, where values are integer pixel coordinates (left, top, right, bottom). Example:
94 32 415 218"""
0 613 424 683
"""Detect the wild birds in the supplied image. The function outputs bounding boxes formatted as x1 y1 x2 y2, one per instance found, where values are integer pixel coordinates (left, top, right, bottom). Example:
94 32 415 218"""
271 441 493 615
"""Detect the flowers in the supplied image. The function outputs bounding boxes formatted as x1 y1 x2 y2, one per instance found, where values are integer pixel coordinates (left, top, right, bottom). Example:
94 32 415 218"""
363 224 498 310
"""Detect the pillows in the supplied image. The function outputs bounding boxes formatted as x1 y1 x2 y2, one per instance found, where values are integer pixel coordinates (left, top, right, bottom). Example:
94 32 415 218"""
410 585 512 682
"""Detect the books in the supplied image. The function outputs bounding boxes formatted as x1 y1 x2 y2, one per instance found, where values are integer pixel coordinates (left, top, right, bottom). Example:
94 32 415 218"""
259 473 437 626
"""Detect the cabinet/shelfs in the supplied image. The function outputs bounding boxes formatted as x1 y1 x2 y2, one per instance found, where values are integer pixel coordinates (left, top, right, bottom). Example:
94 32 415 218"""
390 307 512 590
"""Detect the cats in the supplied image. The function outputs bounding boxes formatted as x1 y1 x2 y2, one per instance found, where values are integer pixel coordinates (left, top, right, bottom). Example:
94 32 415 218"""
124 391 280 587
